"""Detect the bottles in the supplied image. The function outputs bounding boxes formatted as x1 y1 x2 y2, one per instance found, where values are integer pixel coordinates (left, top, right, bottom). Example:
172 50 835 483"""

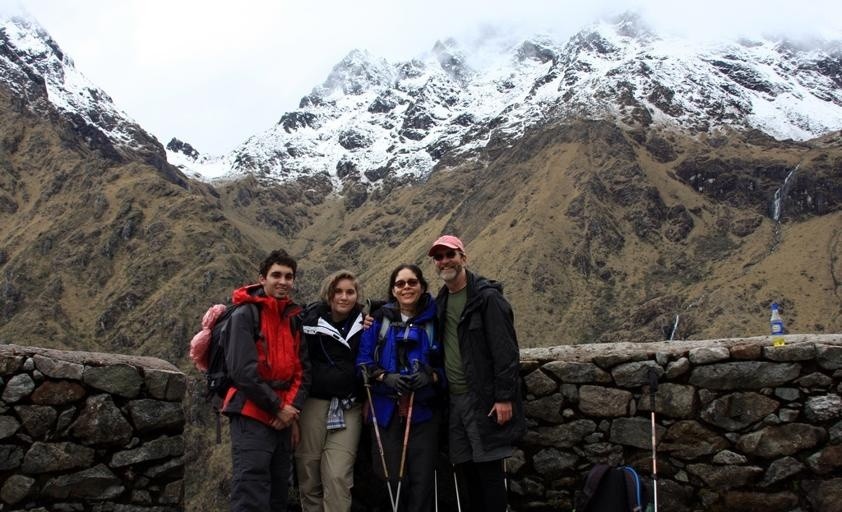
428 235 465 256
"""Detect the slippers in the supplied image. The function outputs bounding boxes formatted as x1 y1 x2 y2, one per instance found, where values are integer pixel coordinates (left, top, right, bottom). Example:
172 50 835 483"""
207 301 296 400
577 465 647 512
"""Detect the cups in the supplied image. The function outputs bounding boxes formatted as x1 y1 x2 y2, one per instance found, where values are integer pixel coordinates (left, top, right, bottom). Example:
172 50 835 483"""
433 252 460 260
394 279 419 288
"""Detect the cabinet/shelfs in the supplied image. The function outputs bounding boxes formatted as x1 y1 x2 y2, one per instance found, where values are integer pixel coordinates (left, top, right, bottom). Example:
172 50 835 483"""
769 303 784 347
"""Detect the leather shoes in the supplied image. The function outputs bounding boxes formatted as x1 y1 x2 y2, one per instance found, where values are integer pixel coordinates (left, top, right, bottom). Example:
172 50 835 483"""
410 372 434 390
382 374 411 392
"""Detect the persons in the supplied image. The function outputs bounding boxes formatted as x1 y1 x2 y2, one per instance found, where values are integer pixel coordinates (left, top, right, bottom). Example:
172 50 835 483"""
357 261 448 511
363 236 528 511
291 267 370 512
221 247 313 511
